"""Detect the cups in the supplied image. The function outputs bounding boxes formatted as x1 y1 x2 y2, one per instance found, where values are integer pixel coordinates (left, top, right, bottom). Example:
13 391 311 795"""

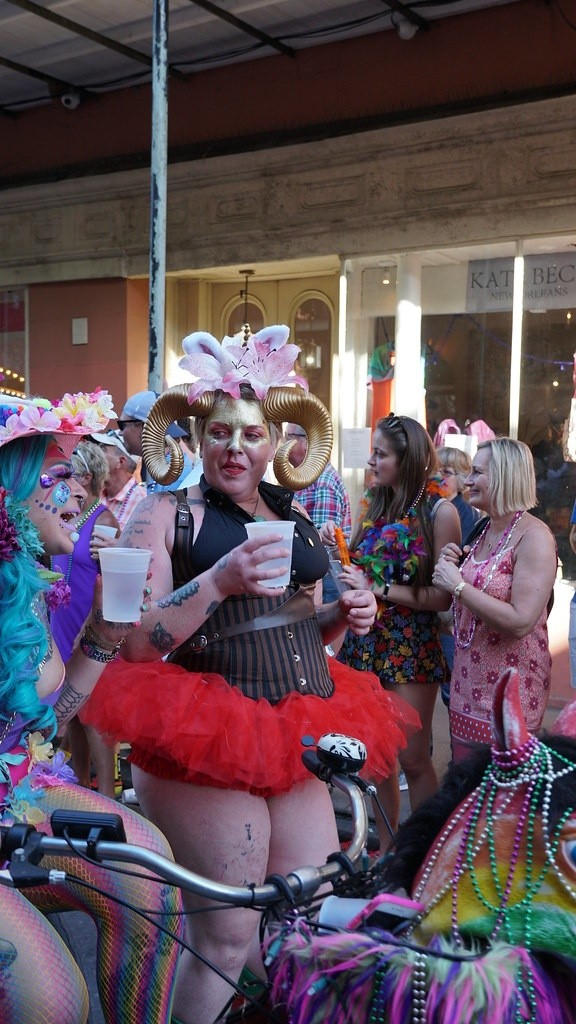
245 521 297 588
92 525 118 542
96 547 153 623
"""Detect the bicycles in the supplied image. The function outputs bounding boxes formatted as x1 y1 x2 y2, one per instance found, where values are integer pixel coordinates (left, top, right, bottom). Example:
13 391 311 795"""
0 730 416 1024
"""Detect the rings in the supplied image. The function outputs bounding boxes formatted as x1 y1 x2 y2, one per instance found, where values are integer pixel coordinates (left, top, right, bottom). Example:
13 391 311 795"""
443 555 446 559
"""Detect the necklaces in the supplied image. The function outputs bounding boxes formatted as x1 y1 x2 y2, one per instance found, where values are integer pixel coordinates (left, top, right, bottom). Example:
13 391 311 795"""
118 483 138 520
454 511 527 651
406 483 426 518
370 732 576 1023
49 498 102 584
247 500 267 522
150 481 156 494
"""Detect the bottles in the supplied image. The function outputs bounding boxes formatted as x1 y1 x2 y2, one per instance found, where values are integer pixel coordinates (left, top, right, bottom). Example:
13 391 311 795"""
335 527 351 570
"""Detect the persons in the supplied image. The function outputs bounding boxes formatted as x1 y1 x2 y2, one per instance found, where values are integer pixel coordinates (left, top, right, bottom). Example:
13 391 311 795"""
432 437 556 763
0 401 182 1023
439 448 478 704
569 498 576 692
64 390 205 799
286 424 353 604
79 382 378 1024
319 416 462 853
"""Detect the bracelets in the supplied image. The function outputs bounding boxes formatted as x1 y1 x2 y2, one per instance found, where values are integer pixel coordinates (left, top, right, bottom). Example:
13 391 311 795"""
382 584 388 599
80 638 116 663
85 626 116 650
454 580 467 596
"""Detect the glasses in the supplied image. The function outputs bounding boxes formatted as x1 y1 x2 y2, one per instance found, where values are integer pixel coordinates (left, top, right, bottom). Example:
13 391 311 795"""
386 412 408 438
73 448 90 473
106 429 131 454
117 419 141 431
285 433 306 440
437 470 457 477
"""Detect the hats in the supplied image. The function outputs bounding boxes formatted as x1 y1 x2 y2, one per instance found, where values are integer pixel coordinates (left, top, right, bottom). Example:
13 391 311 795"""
123 391 188 437
0 386 118 446
90 429 140 464
